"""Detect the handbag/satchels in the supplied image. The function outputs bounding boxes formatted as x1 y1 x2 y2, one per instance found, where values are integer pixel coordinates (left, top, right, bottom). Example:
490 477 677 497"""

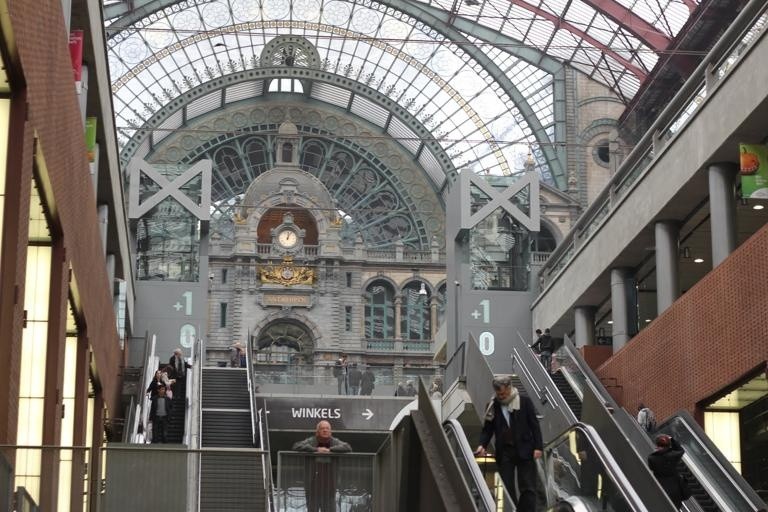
167 390 173 400
146 421 153 441
679 475 691 501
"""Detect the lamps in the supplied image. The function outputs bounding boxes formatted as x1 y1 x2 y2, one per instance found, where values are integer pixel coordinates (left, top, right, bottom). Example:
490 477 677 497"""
419 269 427 295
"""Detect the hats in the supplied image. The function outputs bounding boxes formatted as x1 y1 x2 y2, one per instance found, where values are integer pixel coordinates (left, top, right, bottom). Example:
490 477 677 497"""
230 343 244 352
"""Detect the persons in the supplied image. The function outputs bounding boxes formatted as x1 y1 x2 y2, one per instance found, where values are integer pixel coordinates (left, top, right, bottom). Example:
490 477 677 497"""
539 328 555 374
335 353 348 394
147 385 172 443
636 402 657 435
143 369 168 421
168 348 193 400
573 428 612 511
647 433 687 511
394 377 442 398
359 364 375 395
348 361 363 395
527 328 542 347
476 374 544 511
292 419 351 512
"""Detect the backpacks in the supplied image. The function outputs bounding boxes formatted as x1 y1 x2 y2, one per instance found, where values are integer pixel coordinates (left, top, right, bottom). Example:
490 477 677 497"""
642 409 657 430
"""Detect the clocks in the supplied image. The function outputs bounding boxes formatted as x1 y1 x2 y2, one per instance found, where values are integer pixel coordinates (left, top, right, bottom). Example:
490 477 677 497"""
270 222 303 255
